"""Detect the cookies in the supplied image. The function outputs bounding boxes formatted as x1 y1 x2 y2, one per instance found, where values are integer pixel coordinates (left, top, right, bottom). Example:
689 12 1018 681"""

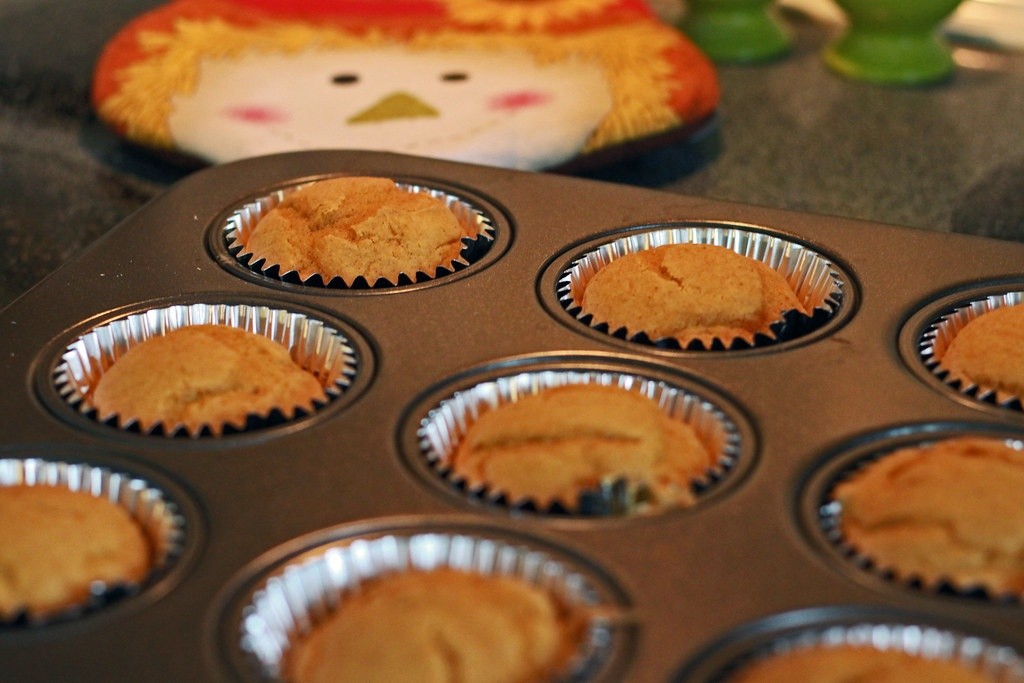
1 174 1023 681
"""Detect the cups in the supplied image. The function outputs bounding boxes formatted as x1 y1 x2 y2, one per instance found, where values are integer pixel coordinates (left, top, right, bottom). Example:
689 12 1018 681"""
672 0 799 63
820 0 963 81
